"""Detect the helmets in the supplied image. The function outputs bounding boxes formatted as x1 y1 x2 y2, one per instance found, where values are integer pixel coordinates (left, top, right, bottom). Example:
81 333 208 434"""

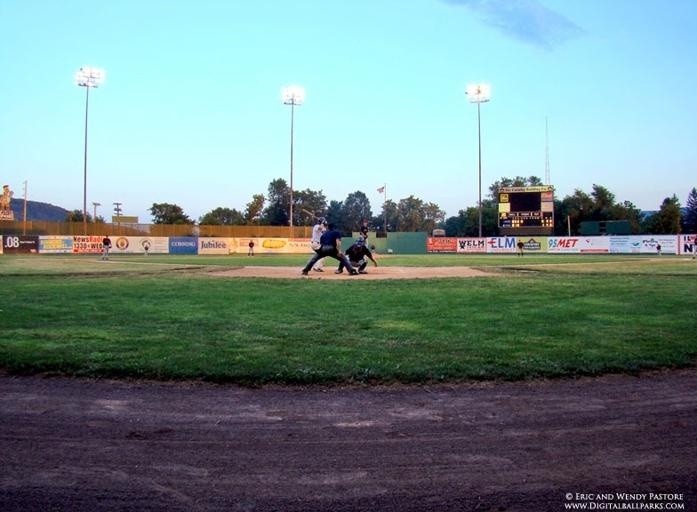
353 236 364 248
318 217 326 224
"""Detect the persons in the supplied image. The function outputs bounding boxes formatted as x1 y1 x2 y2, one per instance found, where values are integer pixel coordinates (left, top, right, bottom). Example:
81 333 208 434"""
309 216 329 272
101 235 112 260
656 243 661 256
517 240 524 255
303 223 358 275
360 219 369 262
693 236 697 259
248 240 254 257
335 236 377 274
145 244 148 255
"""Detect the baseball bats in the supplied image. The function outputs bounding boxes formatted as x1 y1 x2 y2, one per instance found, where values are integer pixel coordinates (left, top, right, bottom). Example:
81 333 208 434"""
301 208 328 224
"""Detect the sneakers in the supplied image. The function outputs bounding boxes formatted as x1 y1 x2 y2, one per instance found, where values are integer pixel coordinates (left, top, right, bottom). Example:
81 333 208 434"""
302 268 322 275
335 269 367 275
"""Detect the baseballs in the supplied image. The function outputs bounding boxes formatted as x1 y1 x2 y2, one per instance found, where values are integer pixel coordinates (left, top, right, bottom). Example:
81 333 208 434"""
388 249 393 253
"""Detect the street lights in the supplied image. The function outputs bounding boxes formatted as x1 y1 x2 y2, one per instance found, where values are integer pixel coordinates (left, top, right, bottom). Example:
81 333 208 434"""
75 65 106 236
465 79 493 237
280 83 305 227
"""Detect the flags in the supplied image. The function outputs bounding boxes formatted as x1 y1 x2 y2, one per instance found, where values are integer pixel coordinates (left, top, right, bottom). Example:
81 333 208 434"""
377 187 384 193
